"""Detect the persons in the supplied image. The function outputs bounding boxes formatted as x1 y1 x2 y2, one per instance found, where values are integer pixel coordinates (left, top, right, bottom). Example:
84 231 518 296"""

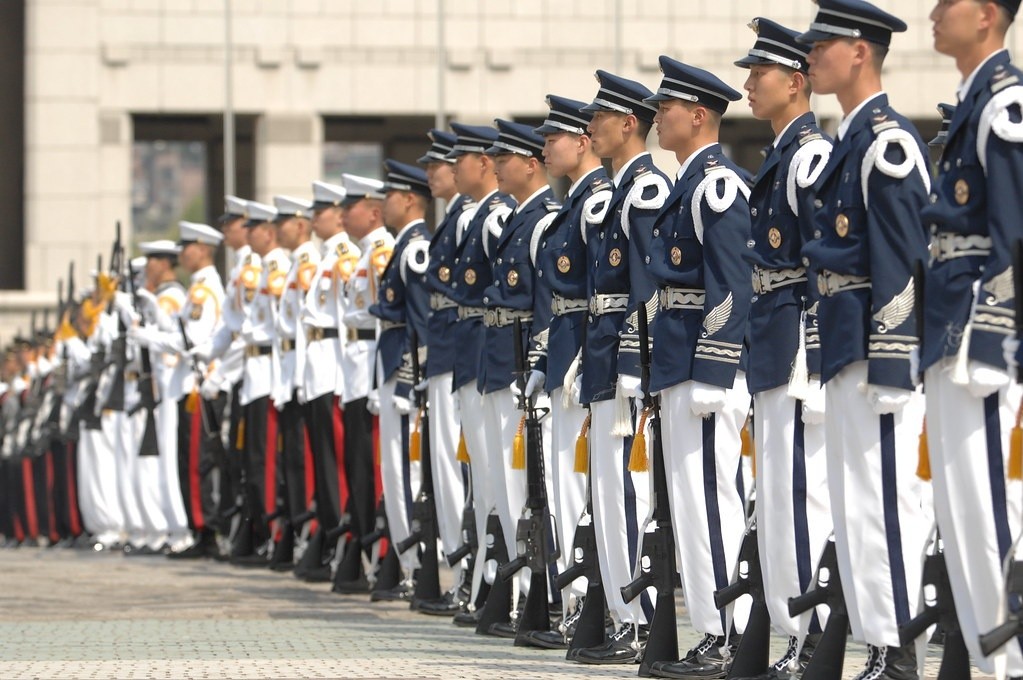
1 69 674 664
796 0 933 680
643 54 752 679
736 18 833 680
918 1 1023 680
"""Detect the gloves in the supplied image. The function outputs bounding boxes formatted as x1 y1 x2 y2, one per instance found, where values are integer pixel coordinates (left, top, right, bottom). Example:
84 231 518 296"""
128 325 1014 420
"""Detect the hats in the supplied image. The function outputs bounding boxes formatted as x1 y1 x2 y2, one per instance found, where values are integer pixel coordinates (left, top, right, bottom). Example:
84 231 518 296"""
175 220 224 247
306 180 346 211
794 0 907 43
416 128 458 164
533 94 595 137
337 173 387 207
579 70 659 124
0 258 147 366
482 118 546 164
270 195 314 224
138 239 185 259
375 158 431 194
444 122 500 159
218 195 250 223
242 200 278 227
642 56 742 116
733 16 812 75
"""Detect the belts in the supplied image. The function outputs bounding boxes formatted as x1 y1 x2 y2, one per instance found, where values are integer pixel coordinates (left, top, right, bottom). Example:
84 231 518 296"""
243 231 995 358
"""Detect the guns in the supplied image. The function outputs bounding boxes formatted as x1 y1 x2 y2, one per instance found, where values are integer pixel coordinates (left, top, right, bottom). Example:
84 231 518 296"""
13 220 1023 680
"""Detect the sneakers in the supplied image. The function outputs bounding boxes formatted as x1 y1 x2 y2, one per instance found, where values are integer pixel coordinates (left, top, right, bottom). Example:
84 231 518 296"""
0 522 919 680
